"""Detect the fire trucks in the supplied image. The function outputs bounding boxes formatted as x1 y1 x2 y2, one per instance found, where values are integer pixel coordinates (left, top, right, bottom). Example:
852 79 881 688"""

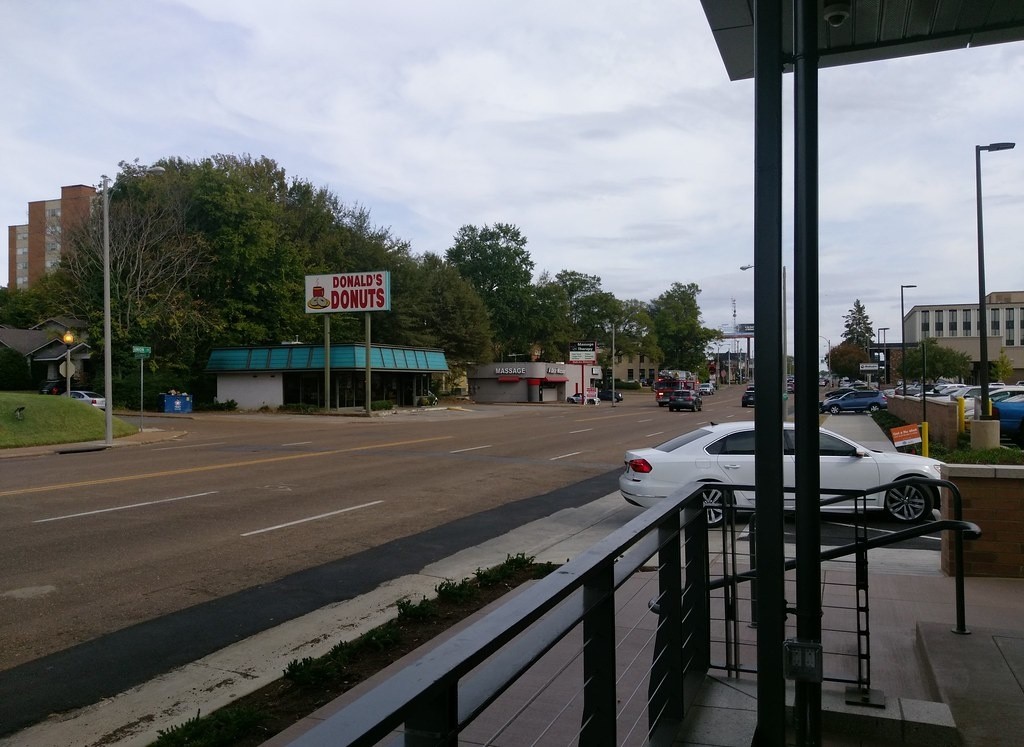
651 370 699 407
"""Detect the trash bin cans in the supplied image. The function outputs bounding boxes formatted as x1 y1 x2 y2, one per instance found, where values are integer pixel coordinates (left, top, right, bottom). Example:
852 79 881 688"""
164 394 192 413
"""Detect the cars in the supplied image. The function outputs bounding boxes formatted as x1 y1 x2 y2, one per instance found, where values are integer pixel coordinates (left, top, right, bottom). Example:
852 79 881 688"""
61 391 105 411
786 373 794 393
883 378 1024 449
818 377 888 415
598 391 623 402
567 393 601 405
619 423 947 531
741 382 755 407
410 389 438 406
640 378 654 386
819 377 829 387
699 380 716 395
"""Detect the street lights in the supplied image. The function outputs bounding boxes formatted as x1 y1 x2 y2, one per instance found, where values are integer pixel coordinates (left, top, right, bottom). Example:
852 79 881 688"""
103 167 167 445
900 285 917 397
716 344 731 384
612 322 636 406
63 330 73 397
878 327 889 389
740 264 788 422
975 143 1015 418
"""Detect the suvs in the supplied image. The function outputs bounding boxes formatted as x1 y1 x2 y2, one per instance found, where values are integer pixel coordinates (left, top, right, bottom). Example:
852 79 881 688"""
668 390 702 412
39 377 78 395
